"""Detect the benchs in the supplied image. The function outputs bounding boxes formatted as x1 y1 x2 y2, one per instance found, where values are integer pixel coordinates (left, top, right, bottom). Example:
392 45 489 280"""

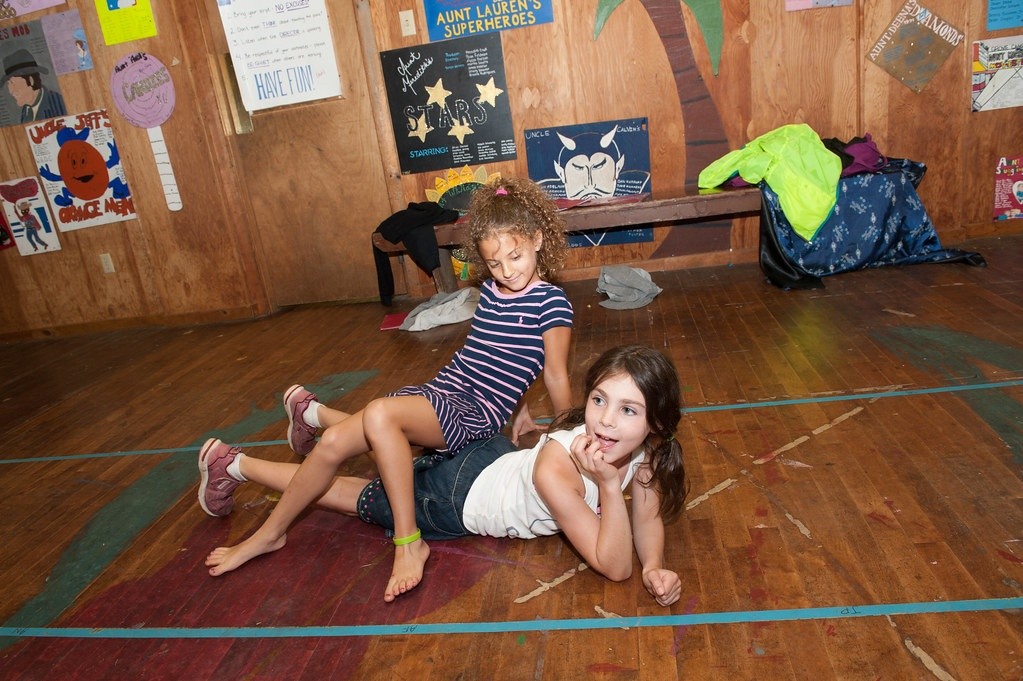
372 172 915 298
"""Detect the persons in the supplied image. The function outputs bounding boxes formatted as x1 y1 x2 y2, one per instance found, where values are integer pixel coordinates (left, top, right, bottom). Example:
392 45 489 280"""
204 175 574 603
197 345 690 607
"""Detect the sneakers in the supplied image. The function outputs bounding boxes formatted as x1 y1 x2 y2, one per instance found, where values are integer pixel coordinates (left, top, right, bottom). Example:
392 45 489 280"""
283 384 318 456
198 437 248 517
